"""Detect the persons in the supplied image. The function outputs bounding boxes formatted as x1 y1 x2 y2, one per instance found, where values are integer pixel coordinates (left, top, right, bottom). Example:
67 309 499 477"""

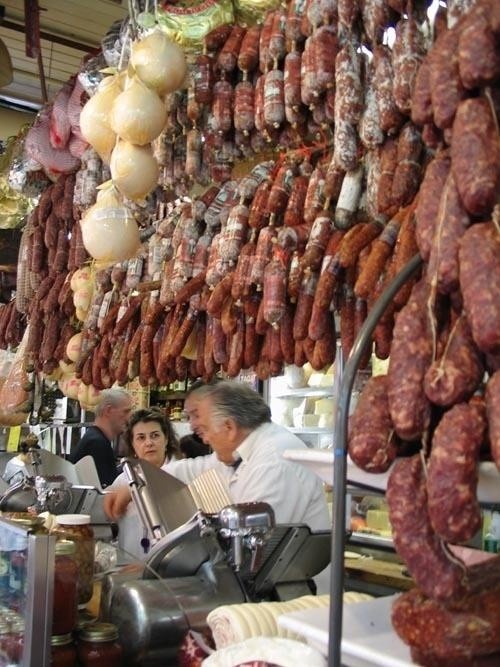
0 439 41 484
71 388 134 490
175 380 220 458
102 381 332 595
102 407 174 572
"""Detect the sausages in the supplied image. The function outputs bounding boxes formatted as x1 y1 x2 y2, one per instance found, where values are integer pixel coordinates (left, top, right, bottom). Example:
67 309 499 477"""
0 1 500 667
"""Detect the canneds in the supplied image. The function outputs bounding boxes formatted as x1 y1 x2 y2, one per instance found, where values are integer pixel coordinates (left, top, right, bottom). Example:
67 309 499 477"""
1 511 125 666
